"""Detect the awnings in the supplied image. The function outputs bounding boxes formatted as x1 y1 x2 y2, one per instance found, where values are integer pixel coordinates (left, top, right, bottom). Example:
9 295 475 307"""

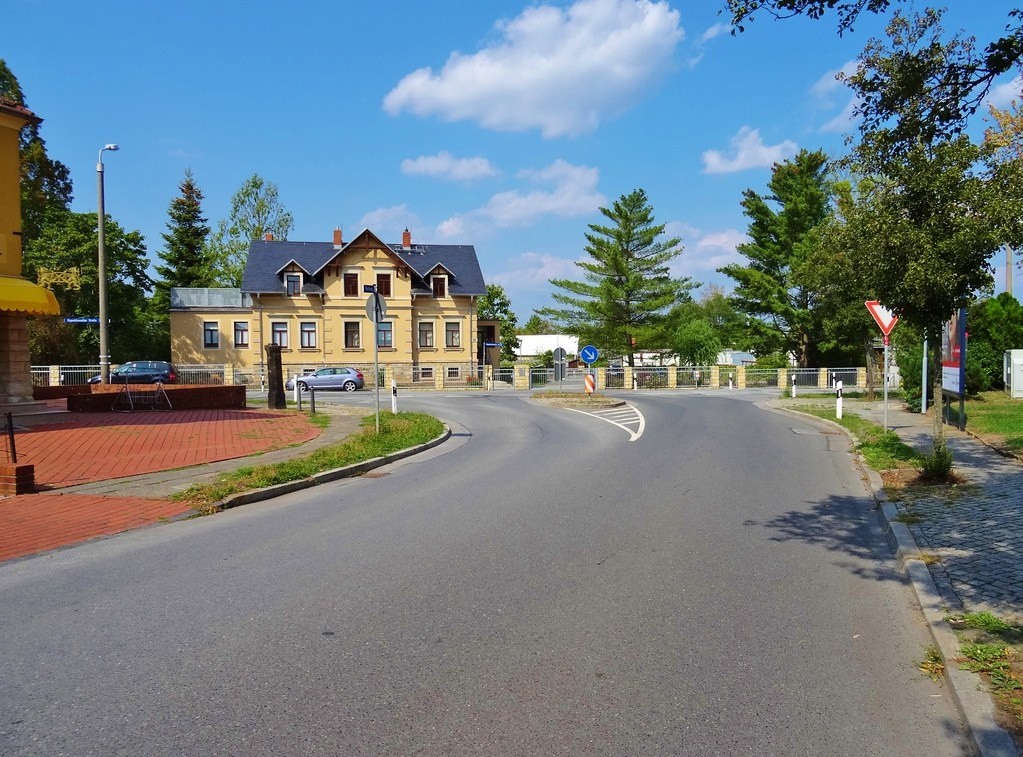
0 276 60 315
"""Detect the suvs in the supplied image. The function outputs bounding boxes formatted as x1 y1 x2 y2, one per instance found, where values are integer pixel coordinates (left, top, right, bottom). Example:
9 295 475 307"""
87 361 181 385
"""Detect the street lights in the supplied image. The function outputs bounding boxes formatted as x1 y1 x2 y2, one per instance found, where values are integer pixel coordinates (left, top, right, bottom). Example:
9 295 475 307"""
96 144 119 384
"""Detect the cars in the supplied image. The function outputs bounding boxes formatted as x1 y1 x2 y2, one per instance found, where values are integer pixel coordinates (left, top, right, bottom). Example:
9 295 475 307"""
286 367 365 393
